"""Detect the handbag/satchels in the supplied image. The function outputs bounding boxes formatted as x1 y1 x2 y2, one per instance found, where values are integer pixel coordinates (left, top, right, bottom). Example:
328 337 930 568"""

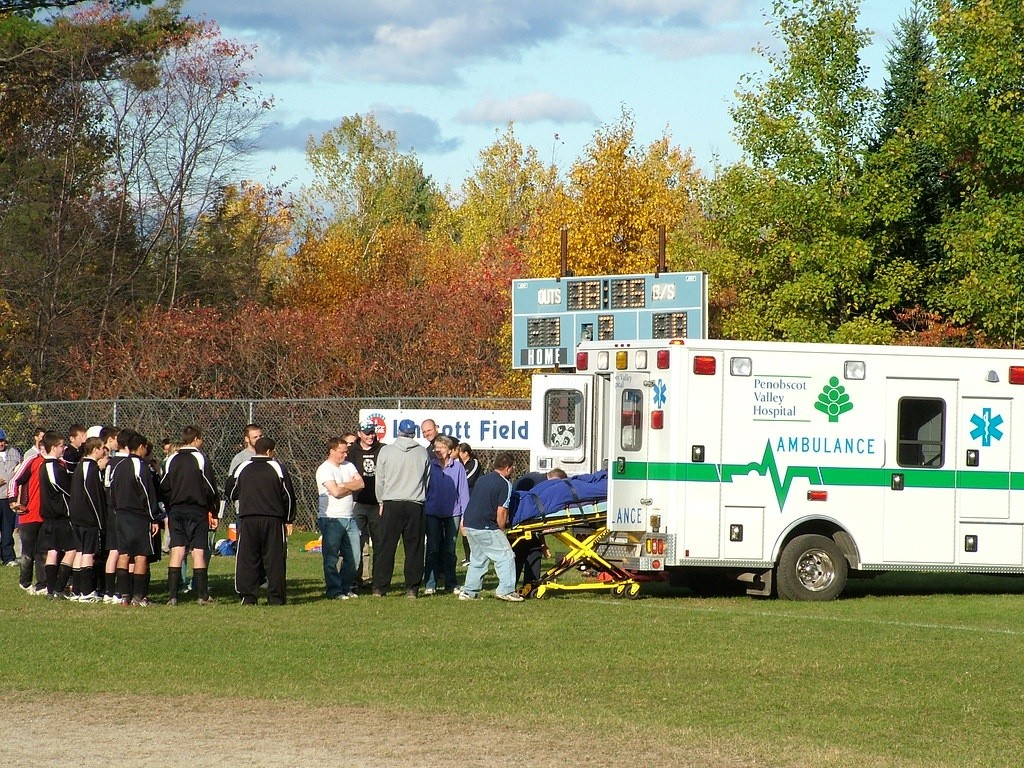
215 539 237 556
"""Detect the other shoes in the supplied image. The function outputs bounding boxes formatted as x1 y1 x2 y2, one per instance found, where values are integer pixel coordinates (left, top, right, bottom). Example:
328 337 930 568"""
334 591 358 600
372 588 386 597
425 589 436 594
407 589 418 599
461 559 470 567
452 587 460 595
17 578 215 608
0 557 18 566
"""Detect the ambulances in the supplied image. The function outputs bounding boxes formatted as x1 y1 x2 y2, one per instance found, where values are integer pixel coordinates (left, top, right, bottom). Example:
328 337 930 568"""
525 335 1024 604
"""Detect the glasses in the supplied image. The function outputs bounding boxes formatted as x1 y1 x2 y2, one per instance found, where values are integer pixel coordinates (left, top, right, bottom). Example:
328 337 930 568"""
347 442 353 445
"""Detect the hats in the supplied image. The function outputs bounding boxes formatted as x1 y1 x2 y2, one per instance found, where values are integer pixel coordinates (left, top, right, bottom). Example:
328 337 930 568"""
359 421 375 435
0 429 6 439
87 426 103 438
399 419 415 434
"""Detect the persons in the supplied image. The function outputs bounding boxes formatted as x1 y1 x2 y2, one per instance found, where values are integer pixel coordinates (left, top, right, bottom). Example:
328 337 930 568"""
0 418 568 608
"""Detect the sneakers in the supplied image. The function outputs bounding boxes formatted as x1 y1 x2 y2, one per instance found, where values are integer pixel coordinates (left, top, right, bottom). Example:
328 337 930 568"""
496 592 524 602
458 592 484 601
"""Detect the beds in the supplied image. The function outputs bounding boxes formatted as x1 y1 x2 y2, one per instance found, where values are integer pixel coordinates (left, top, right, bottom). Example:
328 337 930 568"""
502 501 607 549
